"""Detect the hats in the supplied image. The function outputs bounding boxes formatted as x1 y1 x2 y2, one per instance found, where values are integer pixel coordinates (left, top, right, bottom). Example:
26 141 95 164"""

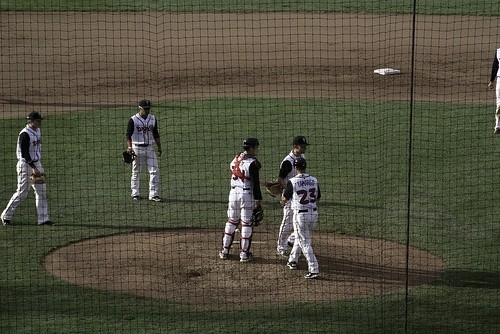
293 136 311 147
138 99 151 108
27 111 45 120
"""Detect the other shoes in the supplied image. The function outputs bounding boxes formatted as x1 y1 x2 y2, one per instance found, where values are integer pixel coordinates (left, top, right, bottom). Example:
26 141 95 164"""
287 240 294 247
1 216 11 226
219 251 227 259
240 252 252 262
277 250 289 258
132 195 139 200
149 196 162 202
40 220 58 226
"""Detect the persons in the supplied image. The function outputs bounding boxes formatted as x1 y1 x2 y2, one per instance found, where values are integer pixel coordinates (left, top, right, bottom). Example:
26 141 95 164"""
219 138 263 262
126 99 162 202
488 48 500 134
276 135 309 257
0 111 53 226
279 158 320 278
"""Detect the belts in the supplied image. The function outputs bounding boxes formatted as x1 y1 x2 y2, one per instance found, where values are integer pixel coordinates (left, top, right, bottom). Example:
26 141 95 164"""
298 208 317 213
135 144 149 147
232 187 251 190
32 160 38 162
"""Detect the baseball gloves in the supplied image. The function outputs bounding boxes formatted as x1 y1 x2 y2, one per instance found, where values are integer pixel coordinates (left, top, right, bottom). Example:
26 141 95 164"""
29 173 46 184
121 150 138 164
249 204 264 228
265 183 284 197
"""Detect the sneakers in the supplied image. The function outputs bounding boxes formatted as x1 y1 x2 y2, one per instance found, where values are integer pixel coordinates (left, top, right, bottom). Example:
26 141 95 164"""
287 261 298 270
304 272 320 279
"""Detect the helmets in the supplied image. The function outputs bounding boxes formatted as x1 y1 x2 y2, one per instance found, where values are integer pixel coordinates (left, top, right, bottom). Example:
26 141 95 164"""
294 157 307 169
242 138 260 147
